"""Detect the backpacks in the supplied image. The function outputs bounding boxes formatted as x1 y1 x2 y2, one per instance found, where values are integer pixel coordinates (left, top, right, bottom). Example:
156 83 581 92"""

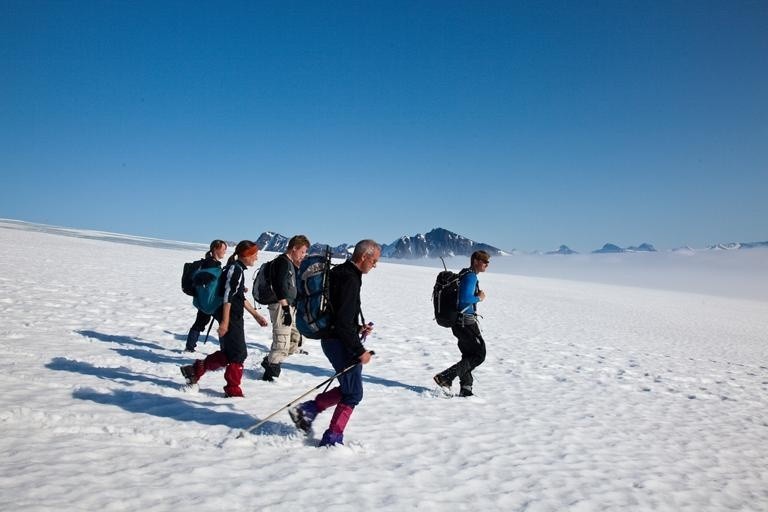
296 254 334 339
252 260 279 305
193 267 223 316
182 258 205 296
434 270 466 327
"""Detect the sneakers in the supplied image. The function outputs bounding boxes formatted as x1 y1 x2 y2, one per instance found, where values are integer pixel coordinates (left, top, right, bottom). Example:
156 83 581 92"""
180 364 198 385
434 373 451 395
459 387 472 397
288 403 312 433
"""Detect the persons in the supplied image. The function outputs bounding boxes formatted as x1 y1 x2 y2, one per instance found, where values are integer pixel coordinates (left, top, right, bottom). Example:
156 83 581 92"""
260 234 310 382
186 238 248 353
433 250 489 398
180 240 267 398
288 239 380 447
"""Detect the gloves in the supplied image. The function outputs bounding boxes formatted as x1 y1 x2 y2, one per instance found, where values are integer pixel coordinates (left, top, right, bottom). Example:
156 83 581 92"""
282 310 291 326
